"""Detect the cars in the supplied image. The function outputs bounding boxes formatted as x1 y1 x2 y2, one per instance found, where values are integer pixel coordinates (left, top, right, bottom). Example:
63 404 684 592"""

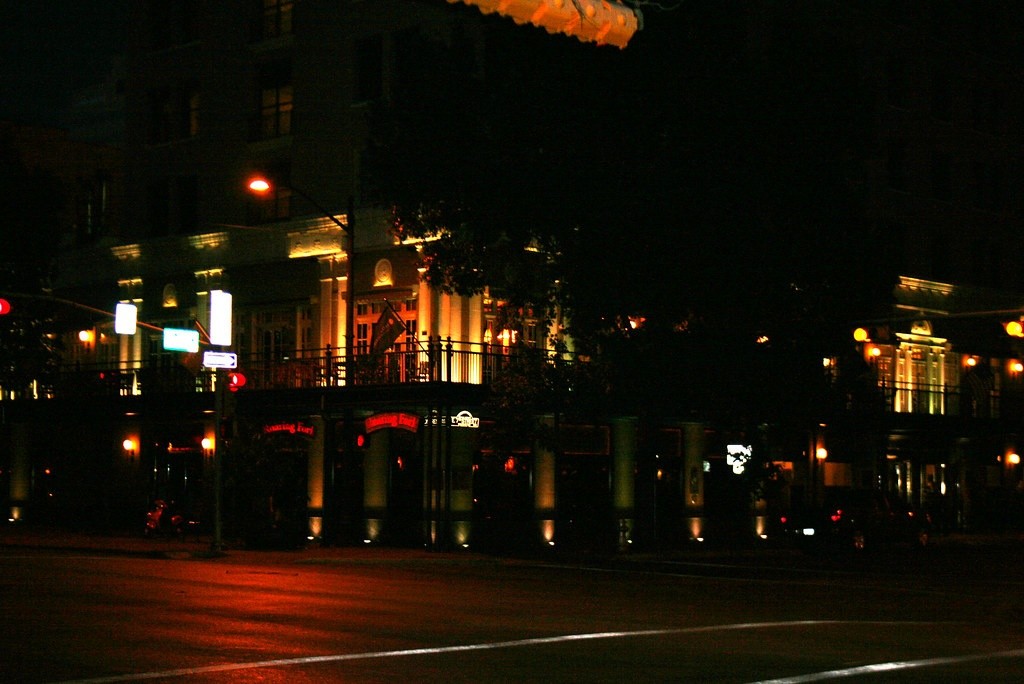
764 483 936 564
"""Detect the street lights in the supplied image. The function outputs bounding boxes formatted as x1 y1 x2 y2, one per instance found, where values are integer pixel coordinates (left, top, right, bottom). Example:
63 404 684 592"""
243 169 354 546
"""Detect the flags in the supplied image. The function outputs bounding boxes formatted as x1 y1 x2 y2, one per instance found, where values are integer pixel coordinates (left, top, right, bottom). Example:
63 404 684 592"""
370 307 403 362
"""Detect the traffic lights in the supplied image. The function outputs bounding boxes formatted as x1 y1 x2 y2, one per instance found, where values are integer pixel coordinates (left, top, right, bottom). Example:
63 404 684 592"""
0 293 49 321
225 369 248 394
97 370 122 382
